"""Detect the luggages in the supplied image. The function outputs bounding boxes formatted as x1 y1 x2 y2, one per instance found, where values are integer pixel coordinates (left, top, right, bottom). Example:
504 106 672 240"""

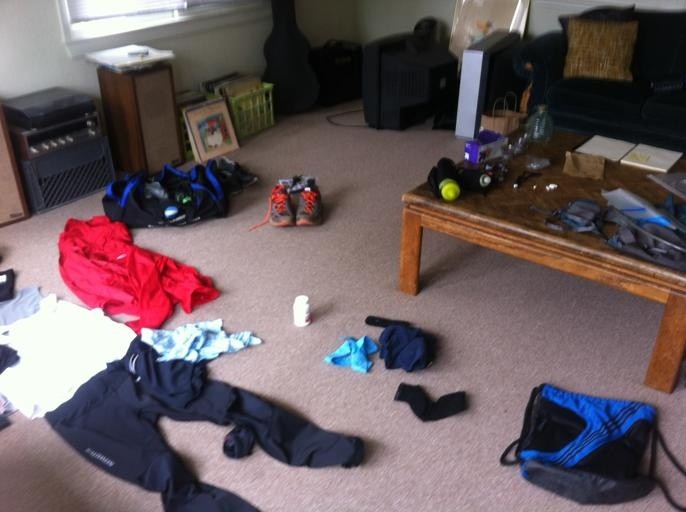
362 34 430 131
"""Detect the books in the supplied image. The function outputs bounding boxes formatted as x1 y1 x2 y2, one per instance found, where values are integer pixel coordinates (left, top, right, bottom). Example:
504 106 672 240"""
574 133 684 174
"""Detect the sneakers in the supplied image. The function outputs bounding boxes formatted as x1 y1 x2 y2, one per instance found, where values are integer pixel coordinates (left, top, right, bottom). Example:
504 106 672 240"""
268 176 322 226
213 156 258 194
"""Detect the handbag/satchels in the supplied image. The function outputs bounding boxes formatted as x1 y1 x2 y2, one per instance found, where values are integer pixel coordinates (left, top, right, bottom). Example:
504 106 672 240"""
515 384 658 507
102 160 229 229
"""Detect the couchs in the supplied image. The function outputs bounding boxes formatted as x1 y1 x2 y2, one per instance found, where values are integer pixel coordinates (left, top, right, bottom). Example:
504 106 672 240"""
517 10 686 156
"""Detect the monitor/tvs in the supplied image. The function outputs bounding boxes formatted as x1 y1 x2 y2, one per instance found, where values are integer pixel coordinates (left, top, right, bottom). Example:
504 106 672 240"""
361 32 458 130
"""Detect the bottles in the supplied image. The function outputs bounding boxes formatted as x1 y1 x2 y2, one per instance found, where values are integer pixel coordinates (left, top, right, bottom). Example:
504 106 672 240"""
526 102 554 151
427 155 493 202
291 294 313 328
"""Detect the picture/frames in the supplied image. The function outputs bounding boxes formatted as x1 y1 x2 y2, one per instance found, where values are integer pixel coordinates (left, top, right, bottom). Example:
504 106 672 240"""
182 96 241 163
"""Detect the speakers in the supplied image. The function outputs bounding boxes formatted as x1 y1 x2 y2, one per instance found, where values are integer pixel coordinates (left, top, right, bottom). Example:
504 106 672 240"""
96 62 187 177
0 101 30 228
20 136 117 214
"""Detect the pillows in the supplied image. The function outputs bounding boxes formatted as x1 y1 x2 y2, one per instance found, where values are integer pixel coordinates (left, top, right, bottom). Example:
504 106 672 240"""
558 6 635 90
563 15 640 81
